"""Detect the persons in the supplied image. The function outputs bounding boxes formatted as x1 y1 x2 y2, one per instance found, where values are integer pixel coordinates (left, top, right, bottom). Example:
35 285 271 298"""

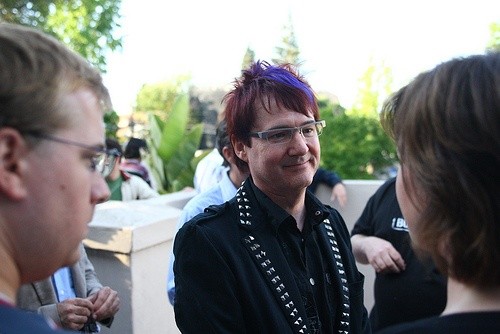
102 136 346 209
350 176 448 333
17 241 120 334
371 52 500 334
1 21 117 334
173 58 367 334
164 117 250 306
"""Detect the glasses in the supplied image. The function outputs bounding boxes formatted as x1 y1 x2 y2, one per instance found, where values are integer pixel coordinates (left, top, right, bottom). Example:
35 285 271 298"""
248 120 329 145
25 129 117 178
